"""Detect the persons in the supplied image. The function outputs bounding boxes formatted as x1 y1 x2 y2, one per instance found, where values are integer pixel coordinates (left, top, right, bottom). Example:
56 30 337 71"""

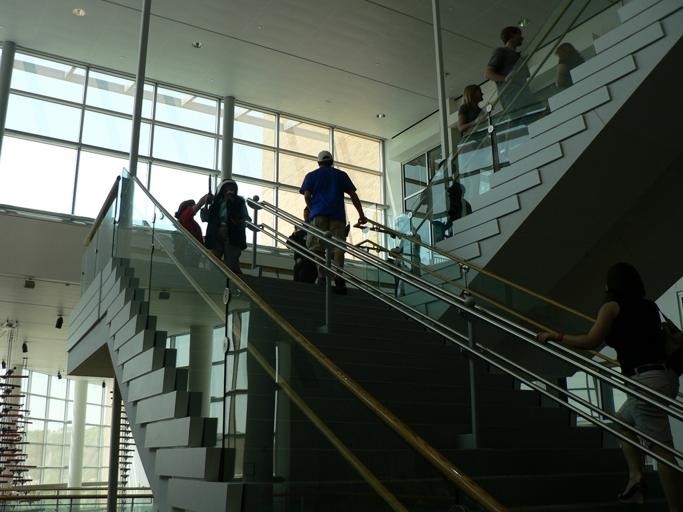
420 158 471 243
484 26 544 137
299 150 367 295
555 42 584 90
534 260 682 511
175 193 212 283
285 207 317 283
200 178 252 297
456 84 488 198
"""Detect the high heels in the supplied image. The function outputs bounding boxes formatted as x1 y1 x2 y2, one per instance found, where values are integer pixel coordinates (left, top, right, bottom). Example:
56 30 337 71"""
615 478 645 508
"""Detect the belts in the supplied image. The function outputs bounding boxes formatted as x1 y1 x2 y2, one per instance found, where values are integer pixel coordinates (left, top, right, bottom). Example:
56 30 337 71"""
619 363 672 378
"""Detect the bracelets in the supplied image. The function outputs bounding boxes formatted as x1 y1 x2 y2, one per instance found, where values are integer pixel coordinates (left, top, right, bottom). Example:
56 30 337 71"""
554 330 564 342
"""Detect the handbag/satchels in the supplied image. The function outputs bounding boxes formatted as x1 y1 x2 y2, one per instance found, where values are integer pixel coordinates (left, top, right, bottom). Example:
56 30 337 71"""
653 302 683 377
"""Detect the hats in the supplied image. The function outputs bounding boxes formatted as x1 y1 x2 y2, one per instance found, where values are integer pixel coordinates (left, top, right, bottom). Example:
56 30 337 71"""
316 150 333 163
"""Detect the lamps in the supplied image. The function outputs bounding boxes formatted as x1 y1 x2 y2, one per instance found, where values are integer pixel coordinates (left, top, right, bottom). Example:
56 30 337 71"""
0 307 108 391
